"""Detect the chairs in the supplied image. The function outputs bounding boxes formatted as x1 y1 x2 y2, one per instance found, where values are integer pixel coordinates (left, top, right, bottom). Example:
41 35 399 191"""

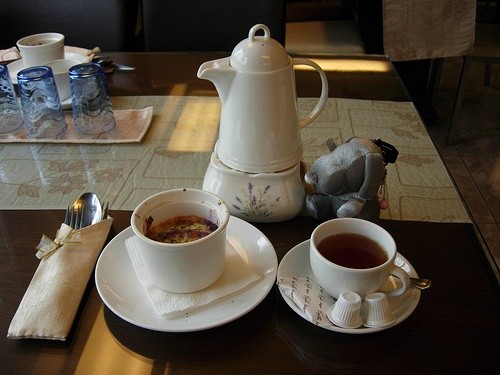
285 0 500 147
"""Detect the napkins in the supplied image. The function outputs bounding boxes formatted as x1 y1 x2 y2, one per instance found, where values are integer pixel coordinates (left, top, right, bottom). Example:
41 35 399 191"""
125 235 261 315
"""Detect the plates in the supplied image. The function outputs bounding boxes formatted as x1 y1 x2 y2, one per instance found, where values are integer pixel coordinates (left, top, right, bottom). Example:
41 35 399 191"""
6 46 91 85
277 240 421 334
95 215 278 333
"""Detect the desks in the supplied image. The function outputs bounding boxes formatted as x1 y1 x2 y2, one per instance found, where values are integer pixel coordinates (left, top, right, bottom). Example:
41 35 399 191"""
0 53 500 375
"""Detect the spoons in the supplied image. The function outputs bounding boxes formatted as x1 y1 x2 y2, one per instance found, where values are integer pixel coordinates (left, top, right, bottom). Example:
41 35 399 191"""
70 191 102 228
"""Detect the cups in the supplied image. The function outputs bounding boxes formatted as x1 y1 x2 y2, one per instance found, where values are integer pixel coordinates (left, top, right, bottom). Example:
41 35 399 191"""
310 218 411 299
130 188 231 294
0 64 23 134
16 33 65 68
16 65 68 137
68 62 116 135
42 59 75 102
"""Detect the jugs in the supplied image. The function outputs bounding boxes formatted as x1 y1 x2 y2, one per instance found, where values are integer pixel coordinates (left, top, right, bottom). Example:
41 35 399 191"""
198 23 329 174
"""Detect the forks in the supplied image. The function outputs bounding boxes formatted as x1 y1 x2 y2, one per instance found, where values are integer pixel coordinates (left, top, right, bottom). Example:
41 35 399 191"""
100 201 109 220
64 205 84 230
